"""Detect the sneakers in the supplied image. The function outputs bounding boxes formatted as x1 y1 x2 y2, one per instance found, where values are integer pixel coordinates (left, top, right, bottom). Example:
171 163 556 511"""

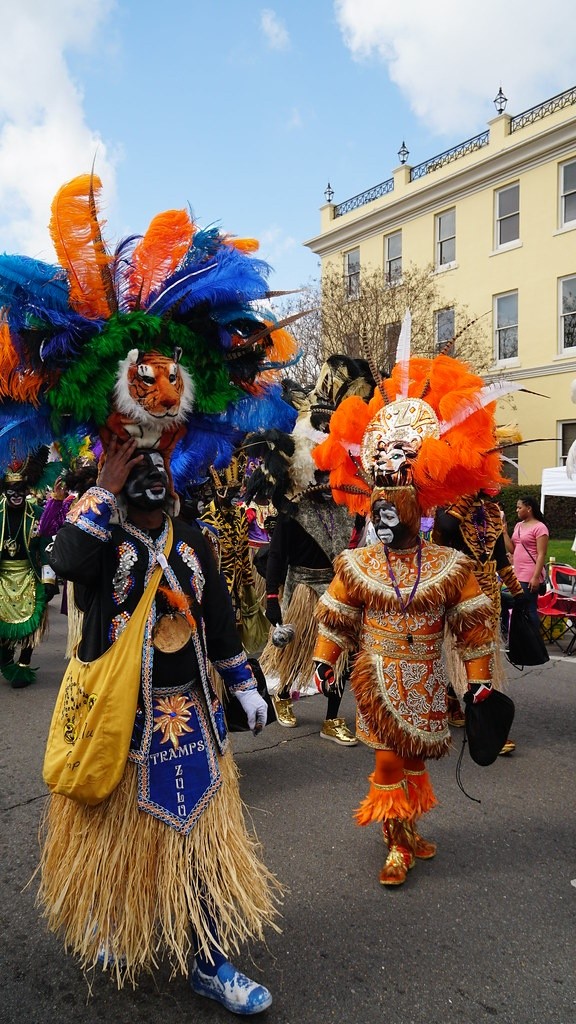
271 692 297 728
321 718 358 746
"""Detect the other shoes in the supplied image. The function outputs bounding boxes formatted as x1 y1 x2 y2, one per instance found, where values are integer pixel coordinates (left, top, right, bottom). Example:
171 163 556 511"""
499 740 515 755
445 687 466 727
84 921 129 967
1 660 37 687
190 959 272 1015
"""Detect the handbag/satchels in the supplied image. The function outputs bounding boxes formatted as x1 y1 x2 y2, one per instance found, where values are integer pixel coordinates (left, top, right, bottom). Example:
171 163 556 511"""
538 582 546 596
238 585 273 655
42 620 146 807
462 689 516 766
507 593 550 671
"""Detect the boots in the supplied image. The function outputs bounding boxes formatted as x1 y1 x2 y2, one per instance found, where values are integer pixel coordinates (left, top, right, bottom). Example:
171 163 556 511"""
383 817 436 859
379 817 417 885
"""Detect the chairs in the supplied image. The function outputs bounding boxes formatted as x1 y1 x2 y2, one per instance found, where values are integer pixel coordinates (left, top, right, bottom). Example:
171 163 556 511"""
535 566 576 655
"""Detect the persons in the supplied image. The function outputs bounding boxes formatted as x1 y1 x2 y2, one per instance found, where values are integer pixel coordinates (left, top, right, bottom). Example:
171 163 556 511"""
266 468 524 885
0 432 277 1015
501 497 549 629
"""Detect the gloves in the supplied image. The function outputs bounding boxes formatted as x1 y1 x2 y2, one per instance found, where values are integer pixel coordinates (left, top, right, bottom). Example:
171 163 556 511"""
314 663 338 697
45 584 54 604
265 597 283 627
468 683 493 704
235 689 268 738
